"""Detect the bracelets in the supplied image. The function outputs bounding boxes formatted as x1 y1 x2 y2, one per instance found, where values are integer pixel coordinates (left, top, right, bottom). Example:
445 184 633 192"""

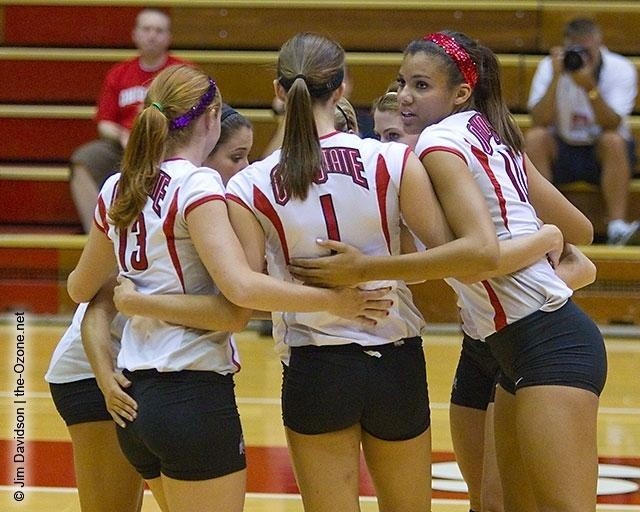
588 88 600 101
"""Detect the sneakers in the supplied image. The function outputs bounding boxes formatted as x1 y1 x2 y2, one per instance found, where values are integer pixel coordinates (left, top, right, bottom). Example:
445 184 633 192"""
607 220 639 246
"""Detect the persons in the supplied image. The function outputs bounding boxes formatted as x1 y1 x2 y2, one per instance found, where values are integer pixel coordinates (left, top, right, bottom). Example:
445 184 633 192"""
64 5 205 237
512 11 638 249
281 28 639 511
263 84 363 165
64 59 399 512
110 26 567 512
40 100 260 511
369 76 599 512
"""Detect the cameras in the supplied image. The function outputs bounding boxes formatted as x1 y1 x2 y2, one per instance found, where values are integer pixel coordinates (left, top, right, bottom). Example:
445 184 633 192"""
559 46 590 71
